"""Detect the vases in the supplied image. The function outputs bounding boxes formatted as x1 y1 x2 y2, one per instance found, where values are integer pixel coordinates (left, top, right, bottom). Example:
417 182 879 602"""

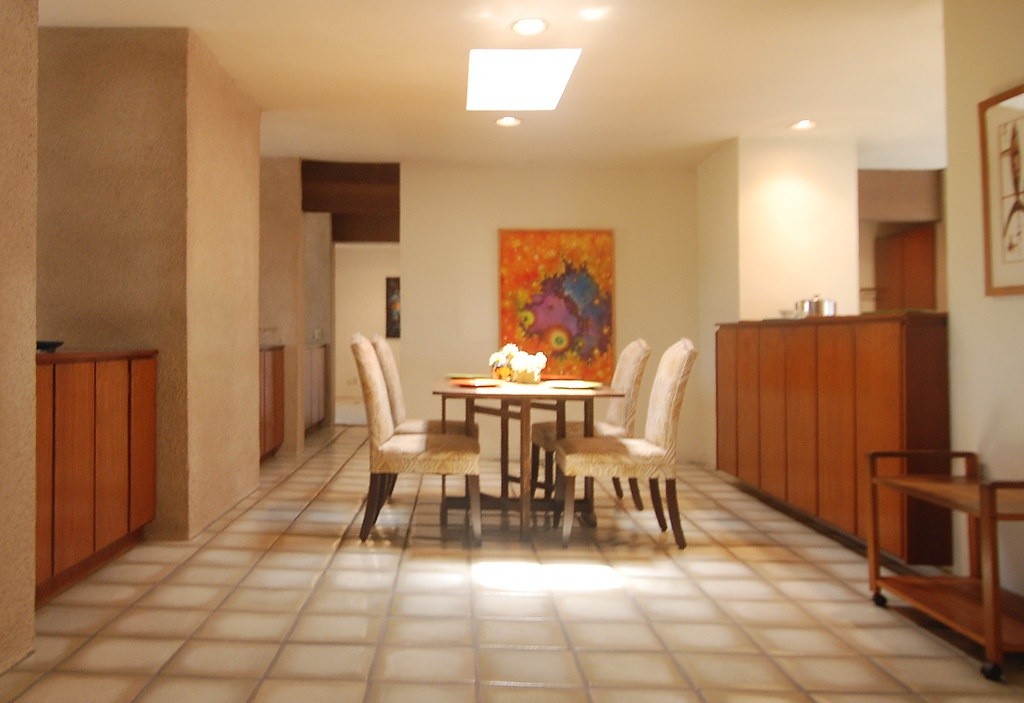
496 367 541 386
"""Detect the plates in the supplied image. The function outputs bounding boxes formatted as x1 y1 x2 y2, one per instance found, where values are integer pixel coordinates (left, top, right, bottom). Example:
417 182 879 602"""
451 379 500 387
547 380 601 389
447 373 492 378
540 374 581 380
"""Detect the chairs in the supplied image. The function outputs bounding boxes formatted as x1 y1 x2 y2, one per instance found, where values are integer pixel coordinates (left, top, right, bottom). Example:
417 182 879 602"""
349 332 483 546
371 333 480 502
551 336 700 549
532 337 652 514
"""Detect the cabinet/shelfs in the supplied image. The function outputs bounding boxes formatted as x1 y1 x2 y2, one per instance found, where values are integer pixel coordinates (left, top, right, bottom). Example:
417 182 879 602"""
304 347 326 430
861 449 1024 679
35 347 158 608
259 341 282 459
714 310 950 564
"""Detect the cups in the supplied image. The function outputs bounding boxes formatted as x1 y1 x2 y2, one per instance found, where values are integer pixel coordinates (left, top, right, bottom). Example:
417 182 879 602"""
823 300 837 317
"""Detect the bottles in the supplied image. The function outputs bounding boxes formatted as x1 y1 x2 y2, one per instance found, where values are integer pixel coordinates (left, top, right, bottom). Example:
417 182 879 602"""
802 299 810 317
814 295 821 315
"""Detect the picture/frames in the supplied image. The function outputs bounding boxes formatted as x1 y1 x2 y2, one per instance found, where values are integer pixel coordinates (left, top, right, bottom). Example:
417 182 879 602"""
978 82 1024 296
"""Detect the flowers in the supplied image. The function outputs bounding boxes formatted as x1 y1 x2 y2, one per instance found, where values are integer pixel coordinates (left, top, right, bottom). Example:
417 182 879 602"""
489 342 547 383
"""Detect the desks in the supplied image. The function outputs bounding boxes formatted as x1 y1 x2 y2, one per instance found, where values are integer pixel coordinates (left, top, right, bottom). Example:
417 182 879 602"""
432 374 626 531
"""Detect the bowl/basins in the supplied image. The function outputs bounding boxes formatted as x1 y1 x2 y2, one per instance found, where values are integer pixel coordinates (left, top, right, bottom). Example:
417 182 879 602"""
37 340 64 353
